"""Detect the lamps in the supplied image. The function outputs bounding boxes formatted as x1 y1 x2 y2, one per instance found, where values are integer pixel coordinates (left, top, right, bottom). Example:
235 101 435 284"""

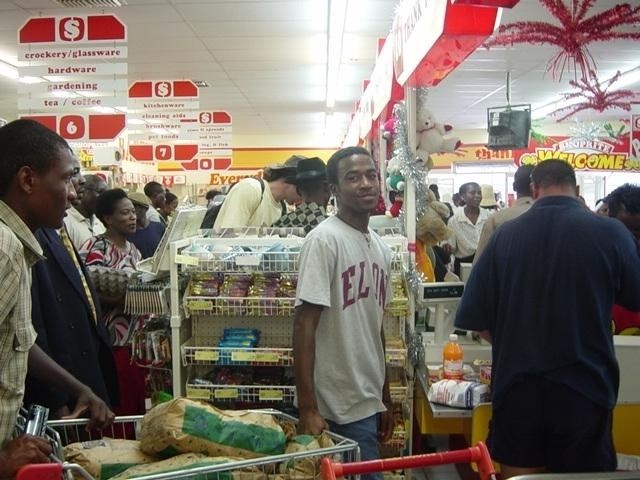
486 70 535 149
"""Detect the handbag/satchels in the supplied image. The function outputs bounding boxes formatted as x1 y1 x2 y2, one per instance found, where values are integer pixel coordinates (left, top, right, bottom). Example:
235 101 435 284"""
200 204 221 230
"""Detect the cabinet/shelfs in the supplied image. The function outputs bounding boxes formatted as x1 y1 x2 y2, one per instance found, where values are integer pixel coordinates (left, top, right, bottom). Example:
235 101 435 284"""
171 231 419 446
128 205 209 409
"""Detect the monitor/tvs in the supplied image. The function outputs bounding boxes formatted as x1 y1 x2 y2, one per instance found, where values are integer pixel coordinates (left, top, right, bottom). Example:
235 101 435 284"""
487 103 532 149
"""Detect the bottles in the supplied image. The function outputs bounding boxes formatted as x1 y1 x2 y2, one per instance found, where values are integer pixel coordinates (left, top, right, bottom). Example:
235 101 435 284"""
443 333 465 381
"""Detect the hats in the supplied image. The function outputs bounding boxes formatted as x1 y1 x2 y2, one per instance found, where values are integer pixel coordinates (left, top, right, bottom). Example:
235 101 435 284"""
127 192 149 208
270 155 326 181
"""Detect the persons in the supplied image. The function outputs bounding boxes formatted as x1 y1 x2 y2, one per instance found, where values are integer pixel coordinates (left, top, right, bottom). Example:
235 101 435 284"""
291 147 394 479
429 163 639 336
0 118 114 480
22 148 122 446
453 159 639 480
201 153 330 236
61 175 179 441
